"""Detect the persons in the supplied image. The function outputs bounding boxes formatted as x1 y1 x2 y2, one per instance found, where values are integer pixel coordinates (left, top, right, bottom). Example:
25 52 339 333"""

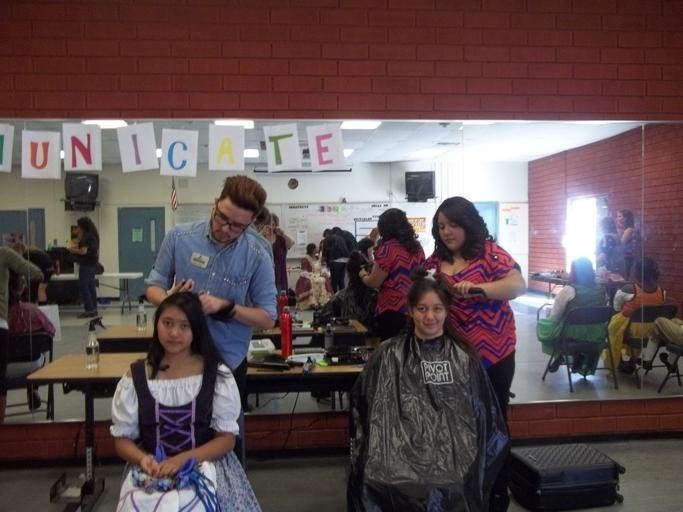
13 241 54 305
145 176 279 470
616 205 641 284
0 246 45 411
5 268 56 379
637 316 682 373
358 208 427 345
422 195 528 426
611 257 665 374
347 277 512 512
68 216 100 318
267 208 381 405
593 218 621 271
252 210 270 236
547 257 607 374
109 292 262 510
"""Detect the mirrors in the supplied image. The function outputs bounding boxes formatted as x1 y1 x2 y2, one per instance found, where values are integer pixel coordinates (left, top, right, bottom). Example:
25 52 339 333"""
0 117 681 427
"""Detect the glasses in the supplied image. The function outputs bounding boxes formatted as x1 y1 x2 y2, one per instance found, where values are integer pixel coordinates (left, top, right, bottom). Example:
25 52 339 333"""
212 201 249 234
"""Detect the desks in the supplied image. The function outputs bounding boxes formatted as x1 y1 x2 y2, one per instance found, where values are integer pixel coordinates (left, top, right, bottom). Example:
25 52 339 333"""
91 316 367 352
528 271 632 319
48 273 144 314
24 354 366 511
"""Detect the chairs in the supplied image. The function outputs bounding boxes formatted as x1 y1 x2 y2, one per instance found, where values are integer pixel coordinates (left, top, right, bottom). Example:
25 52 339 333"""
4 333 54 421
535 304 682 394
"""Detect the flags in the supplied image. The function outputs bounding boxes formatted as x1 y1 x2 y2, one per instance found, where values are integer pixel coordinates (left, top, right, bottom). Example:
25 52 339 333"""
169 180 178 212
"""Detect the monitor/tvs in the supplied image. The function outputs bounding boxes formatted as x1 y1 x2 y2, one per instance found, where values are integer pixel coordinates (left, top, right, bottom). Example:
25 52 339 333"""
405 171 437 198
64 173 98 200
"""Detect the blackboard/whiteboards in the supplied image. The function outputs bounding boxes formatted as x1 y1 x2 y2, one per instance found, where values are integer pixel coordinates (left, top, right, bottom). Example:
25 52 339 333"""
172 201 439 260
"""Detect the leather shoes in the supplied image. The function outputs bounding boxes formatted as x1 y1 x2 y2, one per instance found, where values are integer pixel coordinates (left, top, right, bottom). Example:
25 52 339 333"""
570 354 584 374
549 355 564 374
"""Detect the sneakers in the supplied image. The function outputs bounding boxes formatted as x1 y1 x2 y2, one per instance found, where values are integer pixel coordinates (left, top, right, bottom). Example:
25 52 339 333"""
77 311 99 318
619 352 676 375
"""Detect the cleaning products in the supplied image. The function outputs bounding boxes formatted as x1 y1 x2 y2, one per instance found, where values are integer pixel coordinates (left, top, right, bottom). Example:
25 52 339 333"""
135 294 151 331
83 317 107 370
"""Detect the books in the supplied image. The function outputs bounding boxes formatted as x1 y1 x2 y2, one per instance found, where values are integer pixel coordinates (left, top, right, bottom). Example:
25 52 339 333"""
246 338 275 364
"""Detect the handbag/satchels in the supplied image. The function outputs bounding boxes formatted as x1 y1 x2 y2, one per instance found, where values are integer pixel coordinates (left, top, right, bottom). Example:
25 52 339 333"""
130 444 197 493
